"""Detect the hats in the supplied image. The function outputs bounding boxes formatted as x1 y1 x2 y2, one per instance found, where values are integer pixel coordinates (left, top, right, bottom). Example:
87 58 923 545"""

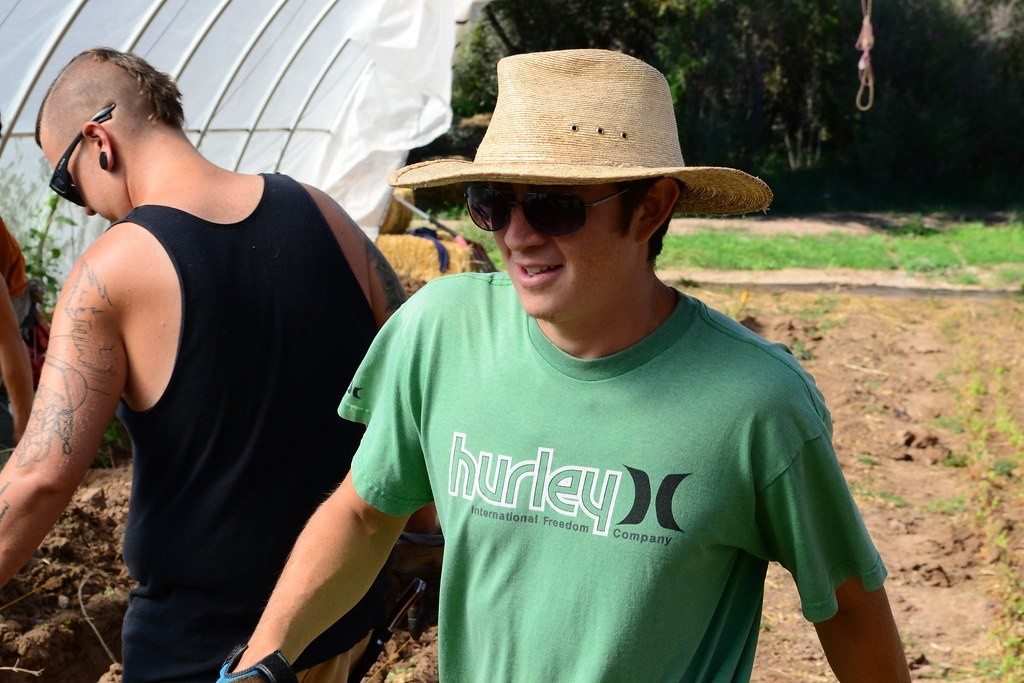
389 49 775 214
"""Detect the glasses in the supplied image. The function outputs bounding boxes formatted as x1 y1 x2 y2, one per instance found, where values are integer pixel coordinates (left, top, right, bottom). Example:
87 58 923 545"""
49 103 116 207
463 185 634 238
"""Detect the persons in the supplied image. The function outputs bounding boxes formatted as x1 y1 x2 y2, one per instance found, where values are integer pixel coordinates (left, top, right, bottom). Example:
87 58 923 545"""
0 217 36 446
1 47 438 683
217 48 911 682
